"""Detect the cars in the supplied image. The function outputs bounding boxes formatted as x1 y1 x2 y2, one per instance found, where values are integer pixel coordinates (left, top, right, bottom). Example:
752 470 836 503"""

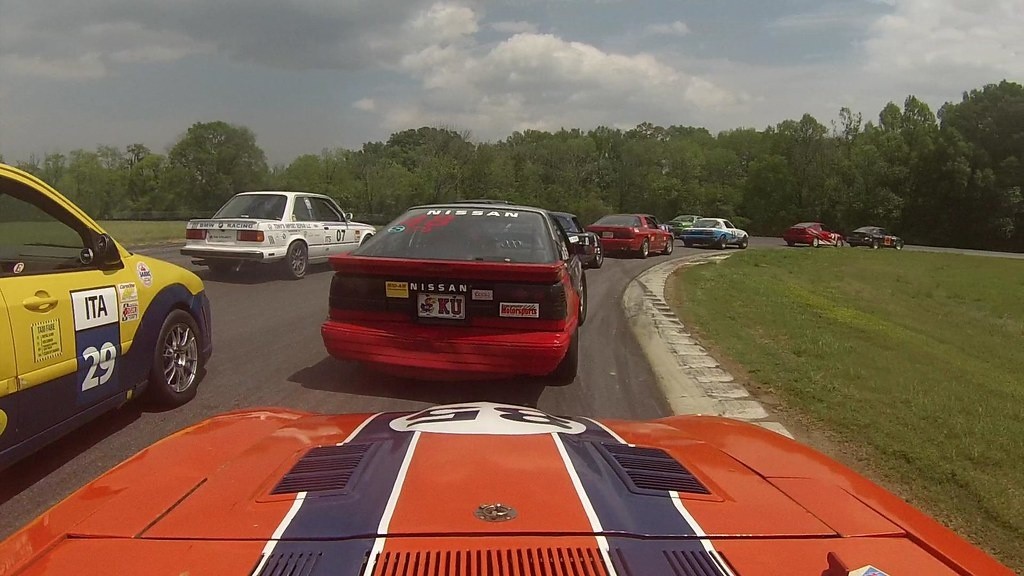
0 402 1019 576
0 163 212 470
584 214 674 259
668 215 703 236
783 223 844 248
320 204 588 390
679 218 749 250
179 191 379 280
648 214 675 240
846 226 905 250
548 211 602 269
